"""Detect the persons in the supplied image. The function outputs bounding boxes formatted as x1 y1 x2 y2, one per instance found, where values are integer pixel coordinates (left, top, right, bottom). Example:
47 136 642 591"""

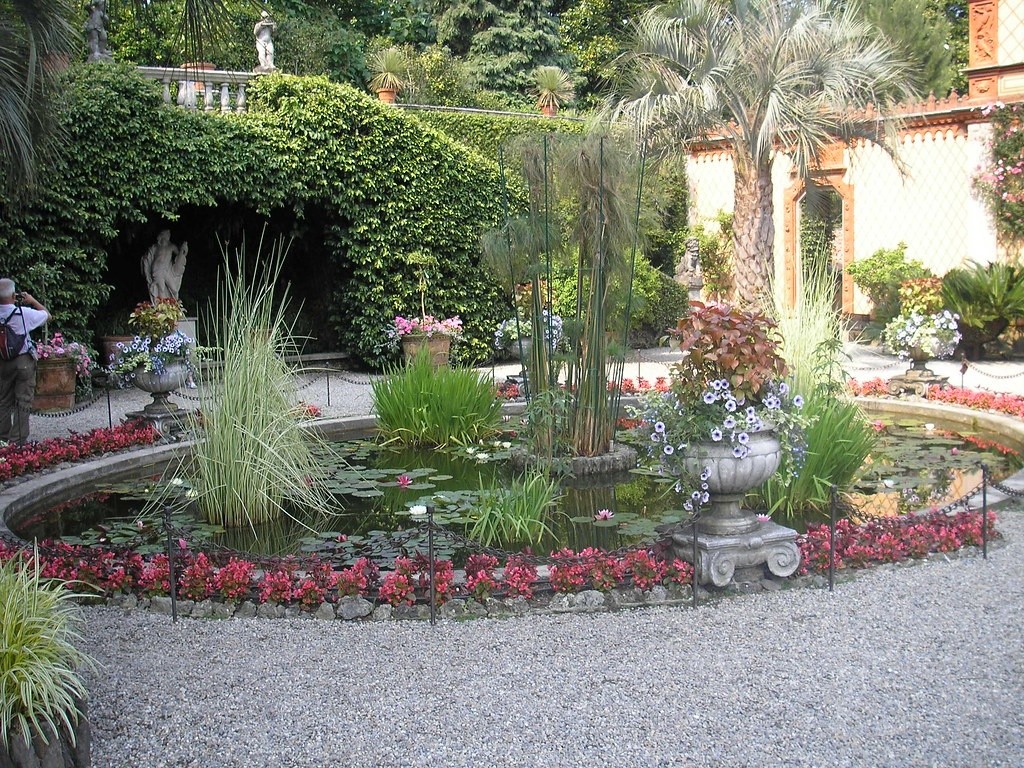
0 279 53 446
147 230 179 298
253 10 276 68
84 4 111 55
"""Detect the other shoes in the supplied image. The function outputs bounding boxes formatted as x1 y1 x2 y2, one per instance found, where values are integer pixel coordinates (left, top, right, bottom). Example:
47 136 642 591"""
18 439 30 446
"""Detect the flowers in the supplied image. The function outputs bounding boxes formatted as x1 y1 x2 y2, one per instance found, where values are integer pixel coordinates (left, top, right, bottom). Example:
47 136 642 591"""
373 315 463 355
36 333 91 377
879 309 962 361
636 300 821 511
108 296 199 390
494 280 564 350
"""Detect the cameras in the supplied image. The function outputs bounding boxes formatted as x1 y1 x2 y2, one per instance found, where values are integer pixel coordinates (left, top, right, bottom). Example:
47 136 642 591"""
14 294 25 302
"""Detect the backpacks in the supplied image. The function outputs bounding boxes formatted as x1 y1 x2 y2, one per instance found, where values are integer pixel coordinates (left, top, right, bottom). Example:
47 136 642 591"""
0 307 27 361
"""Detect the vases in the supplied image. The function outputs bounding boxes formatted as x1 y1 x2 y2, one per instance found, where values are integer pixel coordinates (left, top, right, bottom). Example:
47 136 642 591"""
681 424 782 536
181 63 216 90
905 330 939 377
510 336 550 359
132 357 187 413
402 332 451 372
32 356 76 412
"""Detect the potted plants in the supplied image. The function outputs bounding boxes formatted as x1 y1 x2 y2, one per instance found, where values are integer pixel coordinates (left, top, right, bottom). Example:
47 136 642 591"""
38 0 78 73
94 308 138 365
556 249 663 363
367 48 410 104
530 64 577 116
941 259 1024 361
